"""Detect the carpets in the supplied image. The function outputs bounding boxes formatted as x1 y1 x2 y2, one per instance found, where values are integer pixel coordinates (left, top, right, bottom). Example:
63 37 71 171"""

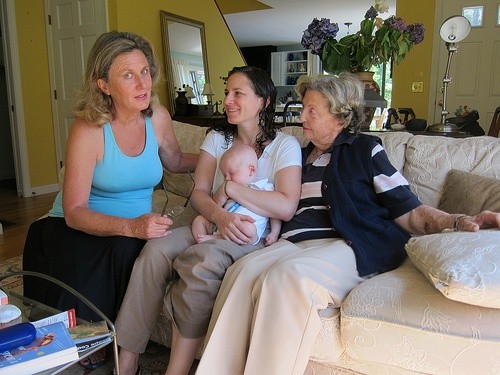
0 252 201 375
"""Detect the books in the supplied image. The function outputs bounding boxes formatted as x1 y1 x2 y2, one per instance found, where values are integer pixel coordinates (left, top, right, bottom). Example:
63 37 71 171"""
0 321 80 375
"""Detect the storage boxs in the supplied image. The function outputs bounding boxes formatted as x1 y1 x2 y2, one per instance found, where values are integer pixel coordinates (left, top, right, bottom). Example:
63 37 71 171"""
186 104 214 117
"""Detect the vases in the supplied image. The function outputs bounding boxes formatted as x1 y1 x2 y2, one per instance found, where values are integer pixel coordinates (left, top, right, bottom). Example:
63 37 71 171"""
176 91 188 115
351 71 380 131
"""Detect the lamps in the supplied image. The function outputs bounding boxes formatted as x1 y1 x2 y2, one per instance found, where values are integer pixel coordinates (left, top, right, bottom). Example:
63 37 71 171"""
184 87 196 104
201 83 215 105
427 15 472 133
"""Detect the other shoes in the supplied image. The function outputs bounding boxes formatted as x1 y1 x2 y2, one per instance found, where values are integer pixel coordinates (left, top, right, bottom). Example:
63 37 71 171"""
80 346 110 370
112 362 143 375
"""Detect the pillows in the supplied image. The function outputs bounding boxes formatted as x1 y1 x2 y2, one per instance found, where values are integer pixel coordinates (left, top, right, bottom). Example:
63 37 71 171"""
406 226 500 309
438 170 500 219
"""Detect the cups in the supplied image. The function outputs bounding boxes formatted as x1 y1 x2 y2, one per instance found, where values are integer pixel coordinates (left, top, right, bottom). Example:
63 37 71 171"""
275 116 299 122
284 96 287 104
288 97 292 102
296 97 301 101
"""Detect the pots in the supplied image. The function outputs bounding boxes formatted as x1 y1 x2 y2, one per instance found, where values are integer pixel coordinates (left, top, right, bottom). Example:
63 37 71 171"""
405 119 427 131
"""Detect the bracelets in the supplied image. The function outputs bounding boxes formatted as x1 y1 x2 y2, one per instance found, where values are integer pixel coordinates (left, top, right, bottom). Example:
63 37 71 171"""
454 215 466 232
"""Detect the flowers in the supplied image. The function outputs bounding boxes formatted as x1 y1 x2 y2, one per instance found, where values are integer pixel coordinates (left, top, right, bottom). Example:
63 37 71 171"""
301 0 426 75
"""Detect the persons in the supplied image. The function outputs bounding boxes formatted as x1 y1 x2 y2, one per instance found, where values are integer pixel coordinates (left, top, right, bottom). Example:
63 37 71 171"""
194 75 500 375
22 31 199 323
191 145 282 247
116 65 302 375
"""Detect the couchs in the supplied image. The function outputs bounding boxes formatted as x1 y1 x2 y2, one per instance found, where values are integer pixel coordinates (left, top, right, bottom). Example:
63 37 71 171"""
59 121 500 375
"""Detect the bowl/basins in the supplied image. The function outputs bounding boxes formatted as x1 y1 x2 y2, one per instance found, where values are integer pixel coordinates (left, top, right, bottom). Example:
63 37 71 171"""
391 124 405 130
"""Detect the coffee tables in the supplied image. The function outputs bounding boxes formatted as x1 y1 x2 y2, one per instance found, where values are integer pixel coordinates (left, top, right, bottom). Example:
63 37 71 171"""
0 272 120 375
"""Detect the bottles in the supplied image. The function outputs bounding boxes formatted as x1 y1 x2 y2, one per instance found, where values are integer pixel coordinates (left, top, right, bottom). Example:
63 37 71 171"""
289 66 294 72
287 77 296 85
175 91 188 105
287 91 297 101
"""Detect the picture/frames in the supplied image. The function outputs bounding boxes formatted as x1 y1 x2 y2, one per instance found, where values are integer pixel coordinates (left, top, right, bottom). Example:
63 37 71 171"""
160 10 213 117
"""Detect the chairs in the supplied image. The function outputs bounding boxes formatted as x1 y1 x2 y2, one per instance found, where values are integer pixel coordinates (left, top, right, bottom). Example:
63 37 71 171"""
386 107 416 129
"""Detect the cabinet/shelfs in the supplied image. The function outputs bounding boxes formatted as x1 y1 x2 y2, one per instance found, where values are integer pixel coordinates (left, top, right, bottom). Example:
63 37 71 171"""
282 50 310 86
270 52 288 85
